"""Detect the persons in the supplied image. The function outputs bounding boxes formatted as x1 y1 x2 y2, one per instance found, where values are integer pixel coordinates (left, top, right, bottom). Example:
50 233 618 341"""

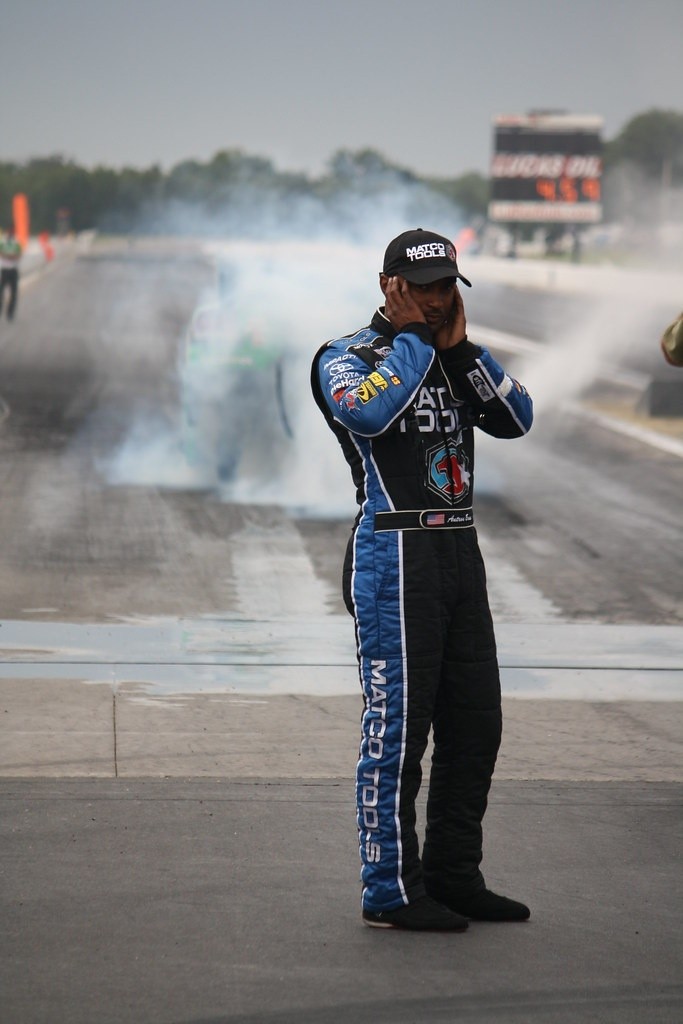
0 225 22 319
309 230 534 933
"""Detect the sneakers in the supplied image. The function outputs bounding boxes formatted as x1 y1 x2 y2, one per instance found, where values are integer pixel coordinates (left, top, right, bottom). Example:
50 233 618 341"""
436 888 530 922
359 895 469 933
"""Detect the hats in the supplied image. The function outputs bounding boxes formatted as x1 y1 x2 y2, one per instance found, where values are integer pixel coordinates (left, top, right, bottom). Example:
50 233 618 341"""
383 227 472 289
